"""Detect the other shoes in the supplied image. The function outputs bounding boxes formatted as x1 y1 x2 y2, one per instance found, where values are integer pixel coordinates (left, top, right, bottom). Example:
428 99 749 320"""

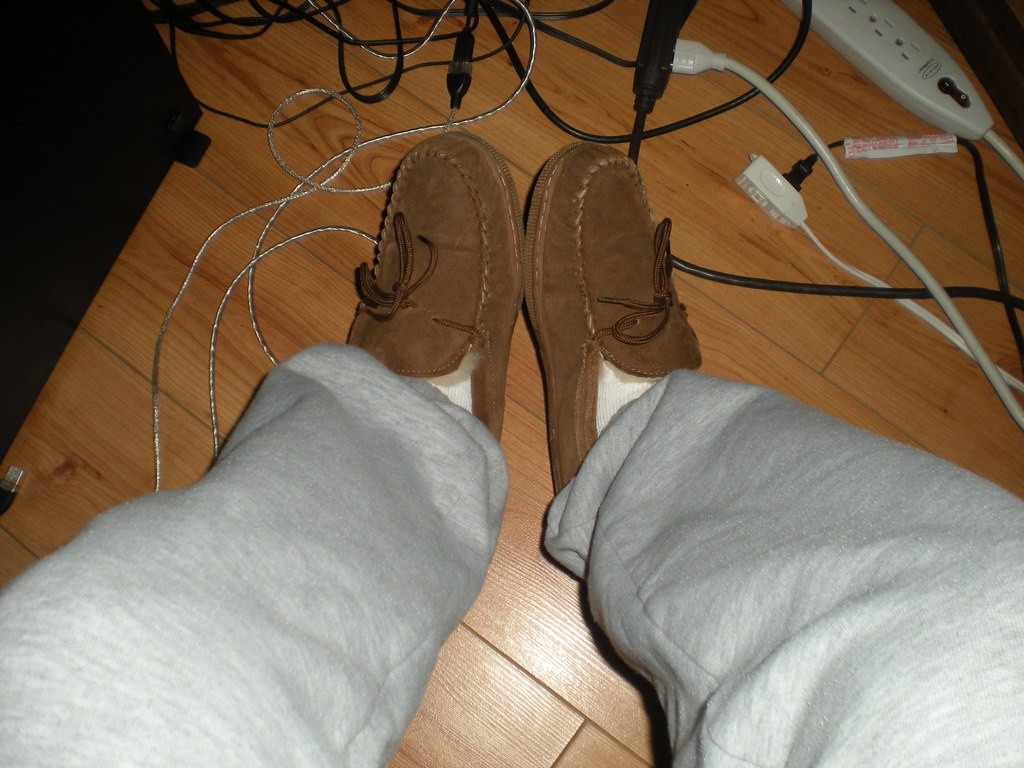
525 139 703 498
346 129 525 444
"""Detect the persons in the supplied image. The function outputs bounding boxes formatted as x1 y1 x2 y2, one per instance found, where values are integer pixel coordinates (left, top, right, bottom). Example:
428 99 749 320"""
1 132 1021 765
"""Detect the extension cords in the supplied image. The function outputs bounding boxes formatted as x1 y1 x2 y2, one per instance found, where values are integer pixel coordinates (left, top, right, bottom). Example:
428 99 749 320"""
777 0 995 142
